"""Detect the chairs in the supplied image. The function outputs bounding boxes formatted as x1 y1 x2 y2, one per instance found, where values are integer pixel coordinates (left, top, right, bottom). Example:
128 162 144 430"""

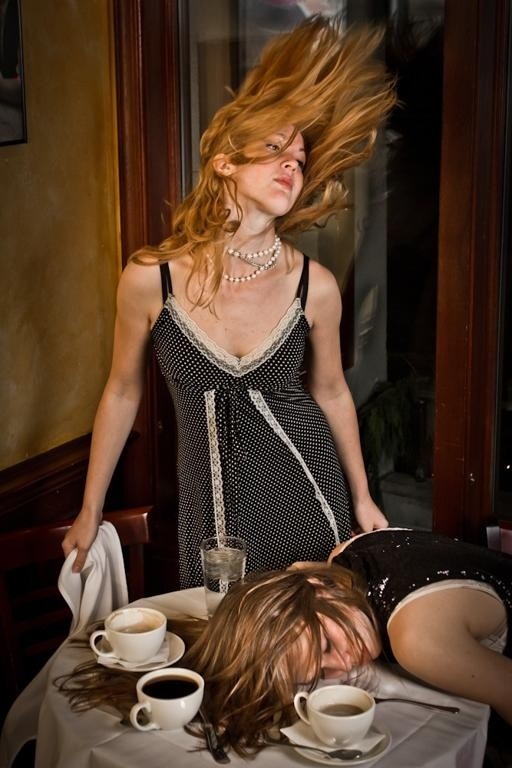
2 504 158 724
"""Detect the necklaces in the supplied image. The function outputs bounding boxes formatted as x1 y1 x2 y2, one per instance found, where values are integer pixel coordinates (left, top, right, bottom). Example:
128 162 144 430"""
241 258 278 270
202 241 282 284
224 234 279 260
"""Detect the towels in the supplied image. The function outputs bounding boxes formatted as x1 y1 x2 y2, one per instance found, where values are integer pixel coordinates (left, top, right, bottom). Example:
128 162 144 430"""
56 515 130 636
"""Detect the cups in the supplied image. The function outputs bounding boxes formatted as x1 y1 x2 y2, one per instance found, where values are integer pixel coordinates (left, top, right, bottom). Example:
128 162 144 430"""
200 537 248 619
89 605 204 733
293 684 376 748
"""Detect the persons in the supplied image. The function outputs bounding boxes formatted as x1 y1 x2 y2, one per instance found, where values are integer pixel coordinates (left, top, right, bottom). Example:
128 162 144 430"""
60 9 393 597
54 526 512 761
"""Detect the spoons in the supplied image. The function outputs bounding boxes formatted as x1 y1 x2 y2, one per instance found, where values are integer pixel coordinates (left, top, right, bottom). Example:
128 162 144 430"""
264 738 364 761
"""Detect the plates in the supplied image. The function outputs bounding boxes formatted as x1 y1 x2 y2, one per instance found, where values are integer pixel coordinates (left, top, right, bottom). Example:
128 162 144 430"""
288 718 392 765
